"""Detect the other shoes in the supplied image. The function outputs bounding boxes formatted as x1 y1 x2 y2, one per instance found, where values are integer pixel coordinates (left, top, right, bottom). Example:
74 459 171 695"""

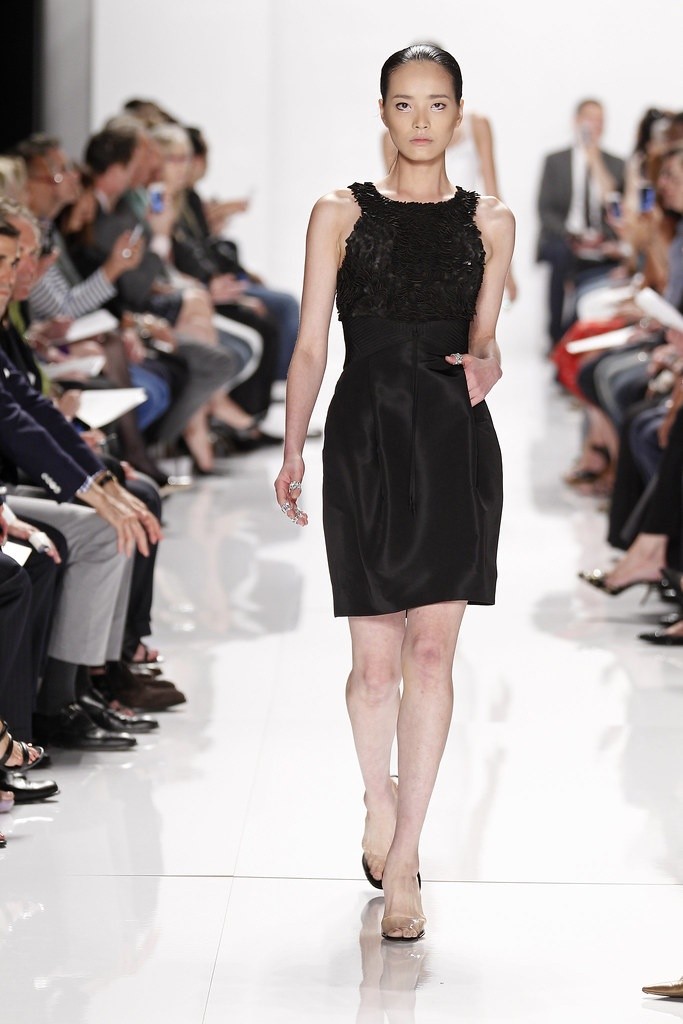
168 419 284 479
565 440 615 484
639 618 683 646
98 663 183 709
126 662 163 678
641 976 683 999
0 789 16 814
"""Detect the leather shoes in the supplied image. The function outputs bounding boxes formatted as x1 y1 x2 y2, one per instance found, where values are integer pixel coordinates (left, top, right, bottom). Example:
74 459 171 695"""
76 693 158 732
47 712 136 751
0 763 58 803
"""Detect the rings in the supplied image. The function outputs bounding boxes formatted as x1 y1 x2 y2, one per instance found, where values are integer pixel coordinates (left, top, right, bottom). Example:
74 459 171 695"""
450 353 463 366
289 480 302 494
281 501 293 514
290 509 304 524
28 531 52 554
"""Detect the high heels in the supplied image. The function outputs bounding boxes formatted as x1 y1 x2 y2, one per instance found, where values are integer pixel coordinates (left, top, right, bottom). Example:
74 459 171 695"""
580 568 666 604
661 567 683 597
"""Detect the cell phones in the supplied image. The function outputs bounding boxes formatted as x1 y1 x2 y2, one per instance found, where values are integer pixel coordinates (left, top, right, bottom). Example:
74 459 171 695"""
123 226 143 257
149 182 165 213
605 191 621 217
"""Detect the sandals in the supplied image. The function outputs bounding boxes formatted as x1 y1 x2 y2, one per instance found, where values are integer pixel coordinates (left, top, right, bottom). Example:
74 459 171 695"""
381 864 426 942
129 642 159 664
361 777 394 890
1 717 44 774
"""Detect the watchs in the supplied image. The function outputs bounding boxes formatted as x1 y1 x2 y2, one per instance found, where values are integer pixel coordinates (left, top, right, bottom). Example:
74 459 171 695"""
97 470 118 486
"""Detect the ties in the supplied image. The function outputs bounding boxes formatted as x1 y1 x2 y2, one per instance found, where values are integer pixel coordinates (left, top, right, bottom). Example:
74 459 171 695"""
584 166 593 227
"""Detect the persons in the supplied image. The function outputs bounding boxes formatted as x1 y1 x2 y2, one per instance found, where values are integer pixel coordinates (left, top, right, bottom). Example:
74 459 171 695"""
0 95 299 852
536 97 683 646
382 116 517 302
275 41 517 943
